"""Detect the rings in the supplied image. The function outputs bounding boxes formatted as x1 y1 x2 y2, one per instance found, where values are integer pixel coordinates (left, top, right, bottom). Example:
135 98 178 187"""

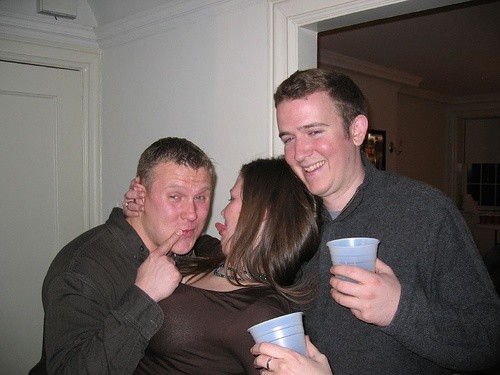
125 200 133 210
266 357 274 370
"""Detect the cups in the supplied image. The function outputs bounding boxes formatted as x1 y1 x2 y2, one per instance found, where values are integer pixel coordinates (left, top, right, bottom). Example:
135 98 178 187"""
247 312 308 358
326 237 380 283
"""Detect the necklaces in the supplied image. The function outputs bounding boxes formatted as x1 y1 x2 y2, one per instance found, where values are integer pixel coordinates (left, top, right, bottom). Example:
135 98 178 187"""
213 261 270 282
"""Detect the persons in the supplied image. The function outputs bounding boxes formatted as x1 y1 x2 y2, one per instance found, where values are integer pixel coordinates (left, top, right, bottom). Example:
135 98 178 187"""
26 137 214 375
272 68 500 375
121 155 332 375
